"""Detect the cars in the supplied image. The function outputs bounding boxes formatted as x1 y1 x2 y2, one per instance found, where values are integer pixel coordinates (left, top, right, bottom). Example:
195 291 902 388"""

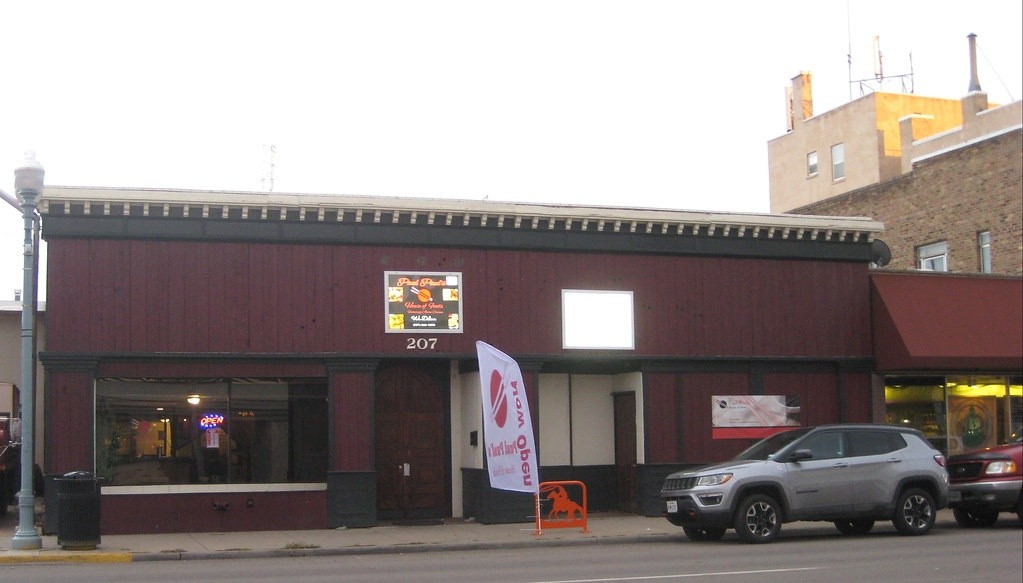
946 425 1022 526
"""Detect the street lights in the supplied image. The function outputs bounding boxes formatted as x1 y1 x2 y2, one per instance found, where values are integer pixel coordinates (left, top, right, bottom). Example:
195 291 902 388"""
13 148 44 550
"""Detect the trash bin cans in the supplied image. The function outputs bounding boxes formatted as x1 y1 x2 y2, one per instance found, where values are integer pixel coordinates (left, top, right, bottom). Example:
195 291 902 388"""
53 470 105 551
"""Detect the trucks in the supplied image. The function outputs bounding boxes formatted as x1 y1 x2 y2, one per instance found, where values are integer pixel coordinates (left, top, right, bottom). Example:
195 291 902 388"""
0 382 23 521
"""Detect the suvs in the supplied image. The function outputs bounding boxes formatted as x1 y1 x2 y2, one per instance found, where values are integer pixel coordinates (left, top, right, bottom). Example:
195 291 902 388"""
660 423 950 544
